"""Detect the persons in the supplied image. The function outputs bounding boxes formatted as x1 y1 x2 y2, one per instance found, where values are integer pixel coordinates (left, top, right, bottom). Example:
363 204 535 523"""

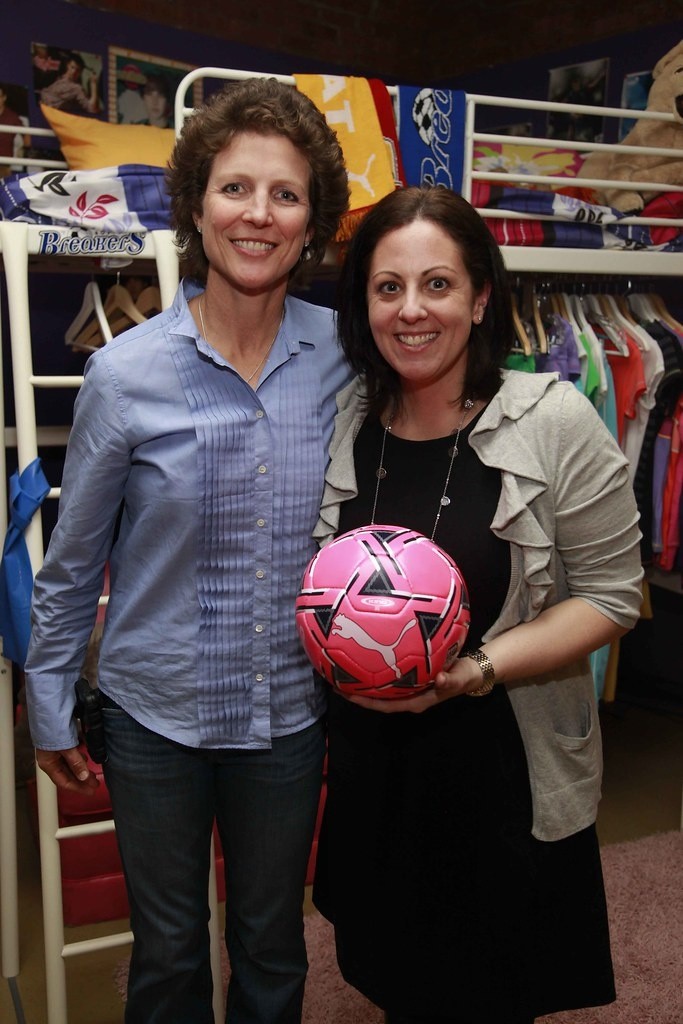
26 78 356 1024
312 189 643 1024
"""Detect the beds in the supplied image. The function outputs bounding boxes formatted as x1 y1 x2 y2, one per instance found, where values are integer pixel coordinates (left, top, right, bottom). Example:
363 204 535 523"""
0 66 682 279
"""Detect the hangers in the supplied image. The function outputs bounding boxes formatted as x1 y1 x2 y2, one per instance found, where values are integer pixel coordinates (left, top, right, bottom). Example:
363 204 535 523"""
62 263 164 352
504 275 683 357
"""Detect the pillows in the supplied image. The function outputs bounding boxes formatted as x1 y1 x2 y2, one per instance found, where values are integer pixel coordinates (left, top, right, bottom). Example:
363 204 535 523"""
36 101 175 171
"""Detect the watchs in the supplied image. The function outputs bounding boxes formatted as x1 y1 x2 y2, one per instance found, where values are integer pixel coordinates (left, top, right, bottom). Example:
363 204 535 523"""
467 649 495 696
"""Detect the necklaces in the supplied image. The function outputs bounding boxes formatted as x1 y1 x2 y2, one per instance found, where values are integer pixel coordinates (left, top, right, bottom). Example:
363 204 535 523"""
198 294 285 383
371 397 475 542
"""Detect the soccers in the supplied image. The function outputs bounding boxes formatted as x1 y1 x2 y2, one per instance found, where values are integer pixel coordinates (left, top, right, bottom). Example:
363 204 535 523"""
294 522 472 700
